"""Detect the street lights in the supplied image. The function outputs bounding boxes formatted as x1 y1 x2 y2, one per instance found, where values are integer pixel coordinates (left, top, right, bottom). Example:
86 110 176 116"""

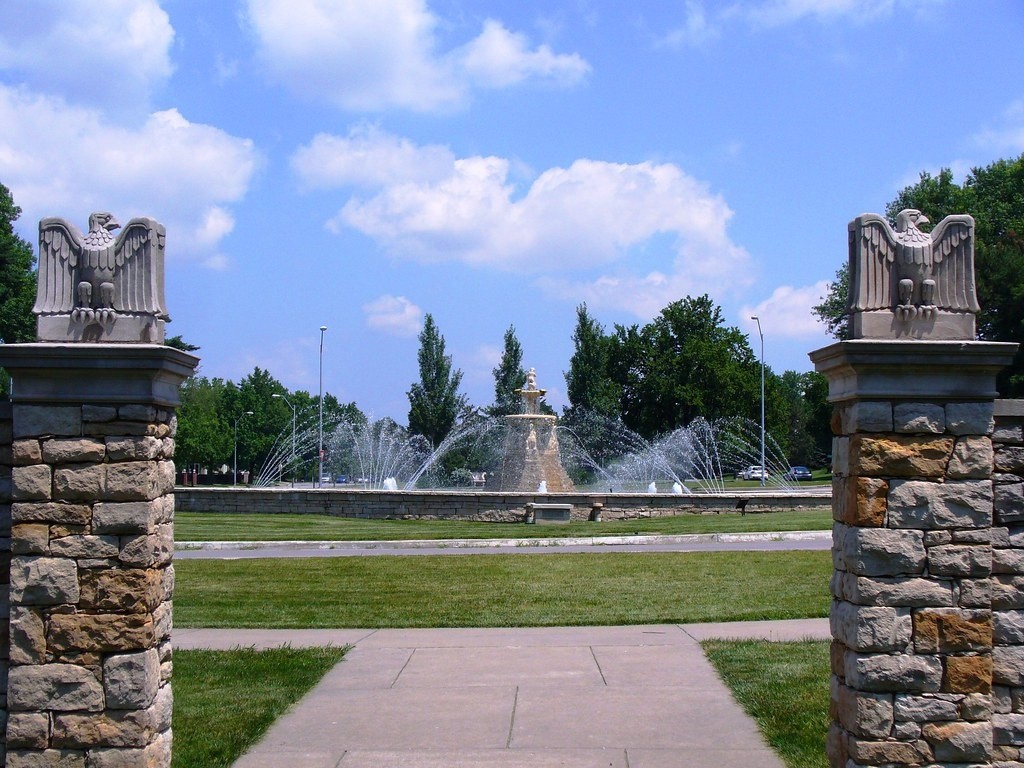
234 412 253 485
750 314 765 487
272 394 295 488
318 326 327 488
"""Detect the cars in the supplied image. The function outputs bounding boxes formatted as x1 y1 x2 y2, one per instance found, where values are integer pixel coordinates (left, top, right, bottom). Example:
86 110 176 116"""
322 473 333 484
735 466 769 481
336 475 375 484
783 466 812 482
